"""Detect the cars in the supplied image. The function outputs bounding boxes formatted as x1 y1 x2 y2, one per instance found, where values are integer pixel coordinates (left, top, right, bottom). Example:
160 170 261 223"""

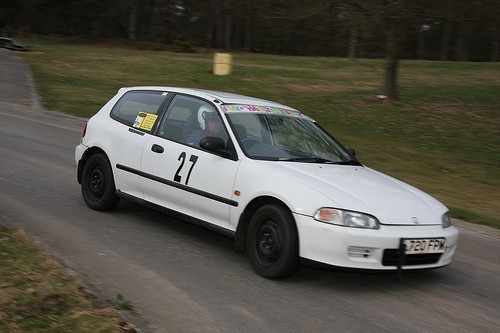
72 83 460 283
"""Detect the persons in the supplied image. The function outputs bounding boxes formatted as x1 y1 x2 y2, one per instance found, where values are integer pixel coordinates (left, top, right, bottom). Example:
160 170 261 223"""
188 104 233 153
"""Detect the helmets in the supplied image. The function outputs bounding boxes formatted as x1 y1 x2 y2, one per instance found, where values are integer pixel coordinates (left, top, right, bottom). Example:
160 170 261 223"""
198 105 223 137
